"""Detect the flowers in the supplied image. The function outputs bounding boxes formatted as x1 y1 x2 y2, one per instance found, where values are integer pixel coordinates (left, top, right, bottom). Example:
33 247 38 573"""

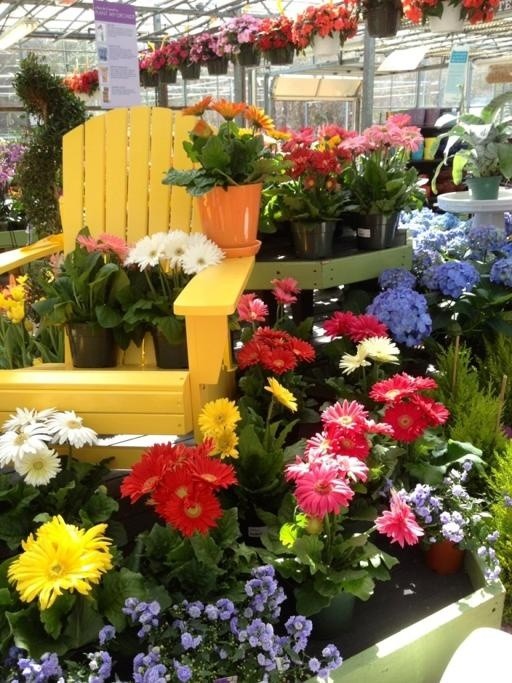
1 0 512 682
